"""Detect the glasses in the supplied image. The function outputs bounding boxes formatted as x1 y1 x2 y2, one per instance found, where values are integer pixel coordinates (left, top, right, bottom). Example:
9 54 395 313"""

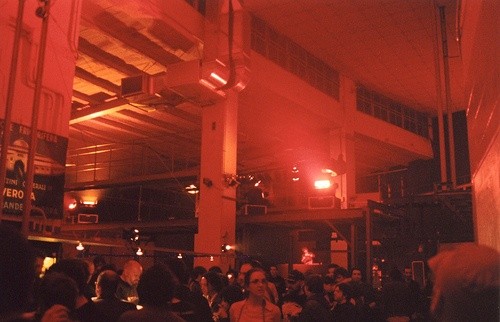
249 279 266 284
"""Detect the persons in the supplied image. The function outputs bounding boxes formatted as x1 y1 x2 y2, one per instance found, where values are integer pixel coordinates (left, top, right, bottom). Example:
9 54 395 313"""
229 267 280 322
428 244 500 322
120 265 187 322
39 257 139 322
157 255 214 322
239 260 274 303
189 264 242 322
270 262 430 322
0 224 40 322
